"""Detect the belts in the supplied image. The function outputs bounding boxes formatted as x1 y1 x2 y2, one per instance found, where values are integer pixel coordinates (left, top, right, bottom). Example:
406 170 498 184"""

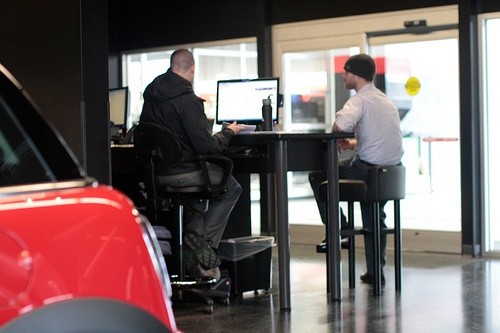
358 159 370 166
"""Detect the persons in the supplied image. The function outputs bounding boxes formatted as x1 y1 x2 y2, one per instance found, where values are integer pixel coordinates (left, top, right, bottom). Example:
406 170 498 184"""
137 49 245 279
308 53 404 289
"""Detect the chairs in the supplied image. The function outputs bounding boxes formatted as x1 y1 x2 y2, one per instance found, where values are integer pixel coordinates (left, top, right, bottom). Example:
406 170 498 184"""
128 123 233 314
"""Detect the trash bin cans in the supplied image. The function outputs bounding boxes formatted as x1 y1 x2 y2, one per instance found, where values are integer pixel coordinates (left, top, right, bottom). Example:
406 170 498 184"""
215 236 273 299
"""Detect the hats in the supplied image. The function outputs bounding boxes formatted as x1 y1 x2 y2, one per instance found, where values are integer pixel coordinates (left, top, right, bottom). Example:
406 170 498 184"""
343 54 376 82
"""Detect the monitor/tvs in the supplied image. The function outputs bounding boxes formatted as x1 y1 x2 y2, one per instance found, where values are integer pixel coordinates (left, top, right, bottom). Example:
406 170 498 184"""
109 87 128 135
216 78 280 124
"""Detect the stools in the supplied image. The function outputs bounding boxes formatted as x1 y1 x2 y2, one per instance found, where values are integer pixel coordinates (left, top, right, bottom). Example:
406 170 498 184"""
319 164 405 296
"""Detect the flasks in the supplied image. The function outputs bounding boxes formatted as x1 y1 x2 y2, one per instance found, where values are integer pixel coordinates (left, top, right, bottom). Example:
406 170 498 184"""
262 98 272 131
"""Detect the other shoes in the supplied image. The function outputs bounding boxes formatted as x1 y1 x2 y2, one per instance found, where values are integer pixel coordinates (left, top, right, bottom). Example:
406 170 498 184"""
197 262 221 282
316 227 349 251
360 271 386 285
184 230 221 269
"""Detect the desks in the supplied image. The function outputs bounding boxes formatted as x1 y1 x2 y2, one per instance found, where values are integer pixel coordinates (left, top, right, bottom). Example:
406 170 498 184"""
226 131 346 310
